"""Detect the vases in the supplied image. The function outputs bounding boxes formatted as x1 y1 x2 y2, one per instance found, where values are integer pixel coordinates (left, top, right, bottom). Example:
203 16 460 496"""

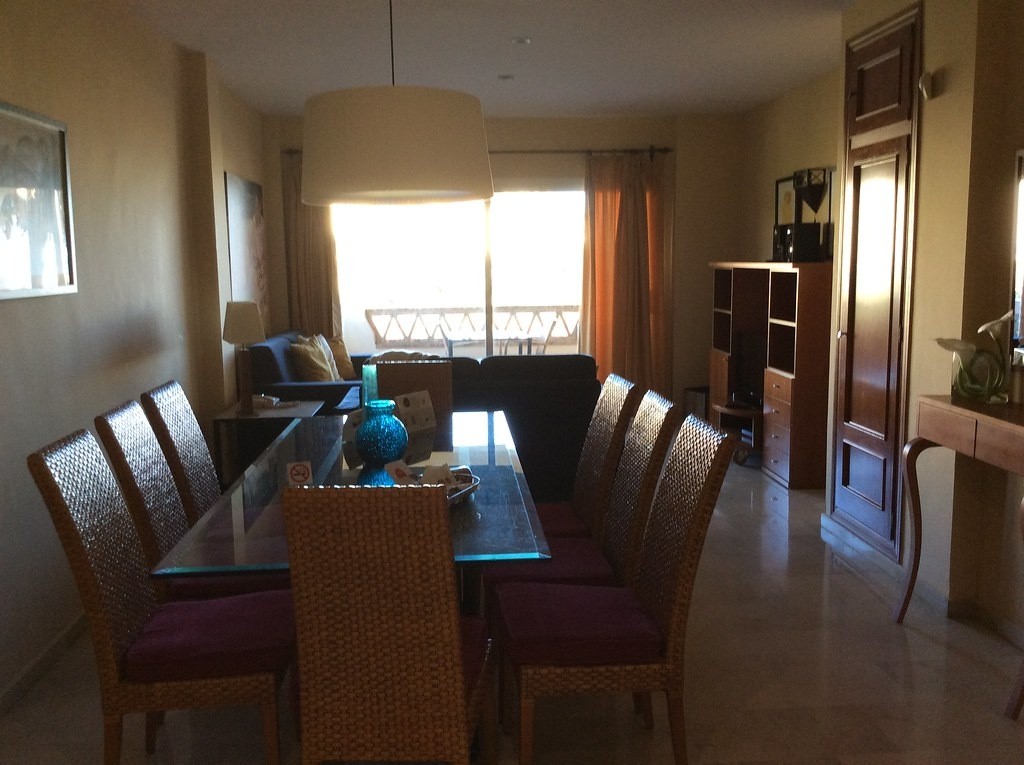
357 398 407 464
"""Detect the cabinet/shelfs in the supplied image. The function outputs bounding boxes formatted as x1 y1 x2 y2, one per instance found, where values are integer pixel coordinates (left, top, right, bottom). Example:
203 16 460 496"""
710 259 828 490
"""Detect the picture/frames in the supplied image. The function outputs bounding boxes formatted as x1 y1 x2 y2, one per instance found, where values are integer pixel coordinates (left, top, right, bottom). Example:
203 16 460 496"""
1 102 80 300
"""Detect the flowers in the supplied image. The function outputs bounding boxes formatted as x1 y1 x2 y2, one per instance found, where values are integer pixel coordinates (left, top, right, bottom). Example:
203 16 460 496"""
936 310 1018 406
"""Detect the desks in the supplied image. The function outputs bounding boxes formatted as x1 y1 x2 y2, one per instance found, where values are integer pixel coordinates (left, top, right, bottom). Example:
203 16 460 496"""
150 409 551 625
214 398 327 489
895 394 1024 722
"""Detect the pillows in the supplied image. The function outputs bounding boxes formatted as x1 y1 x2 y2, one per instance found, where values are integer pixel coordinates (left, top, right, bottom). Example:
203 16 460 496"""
290 334 348 381
297 335 360 381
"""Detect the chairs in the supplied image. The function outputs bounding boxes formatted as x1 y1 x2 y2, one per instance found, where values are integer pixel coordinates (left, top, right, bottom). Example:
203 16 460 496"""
138 380 220 515
28 427 301 765
97 401 286 725
502 414 740 765
471 391 682 635
537 373 637 535
281 486 498 765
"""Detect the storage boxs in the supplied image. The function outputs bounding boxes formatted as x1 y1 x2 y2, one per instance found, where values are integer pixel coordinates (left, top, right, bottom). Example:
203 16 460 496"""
376 359 454 451
390 390 438 465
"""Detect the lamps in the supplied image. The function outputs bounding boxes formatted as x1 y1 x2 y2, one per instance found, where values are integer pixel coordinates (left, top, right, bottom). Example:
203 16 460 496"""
223 301 265 417
301 1 495 206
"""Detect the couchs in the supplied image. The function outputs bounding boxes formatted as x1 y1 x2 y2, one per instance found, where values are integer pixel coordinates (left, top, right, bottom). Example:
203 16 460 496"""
252 329 373 417
363 356 601 480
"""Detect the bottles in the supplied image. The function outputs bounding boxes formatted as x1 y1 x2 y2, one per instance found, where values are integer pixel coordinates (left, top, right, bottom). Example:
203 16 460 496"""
356 399 409 463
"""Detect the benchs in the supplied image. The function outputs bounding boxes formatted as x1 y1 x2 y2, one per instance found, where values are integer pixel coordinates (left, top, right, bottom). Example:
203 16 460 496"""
446 331 537 357
